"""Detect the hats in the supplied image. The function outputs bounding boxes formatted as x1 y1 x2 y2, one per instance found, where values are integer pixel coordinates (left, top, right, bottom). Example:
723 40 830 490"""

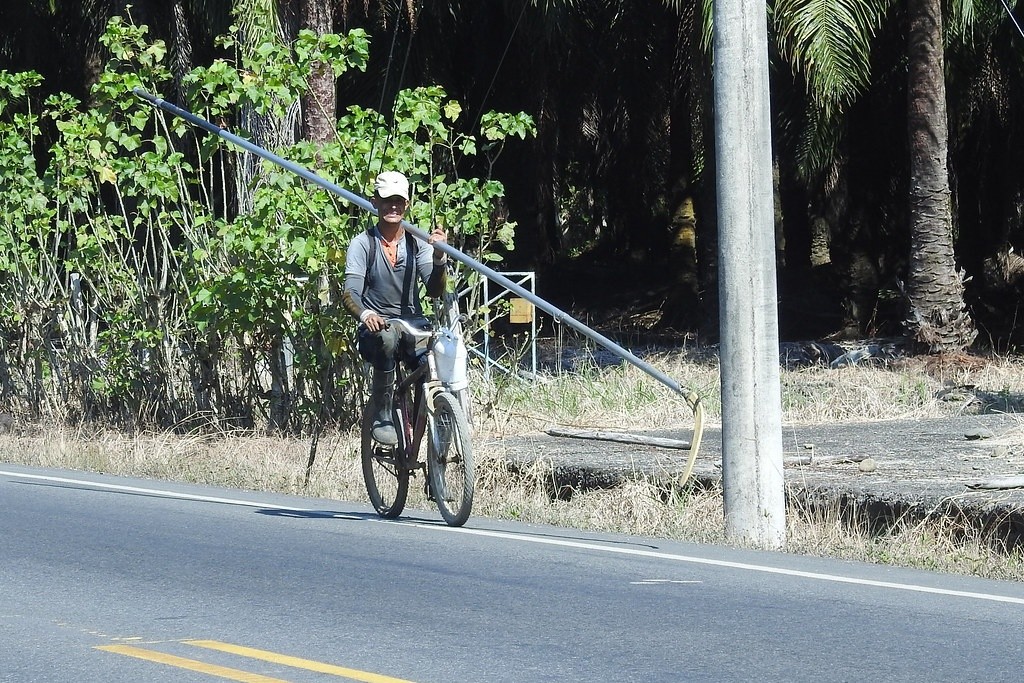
374 170 409 202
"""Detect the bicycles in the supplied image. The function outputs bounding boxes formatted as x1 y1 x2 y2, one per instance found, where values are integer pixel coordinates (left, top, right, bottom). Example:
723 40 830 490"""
359 314 479 528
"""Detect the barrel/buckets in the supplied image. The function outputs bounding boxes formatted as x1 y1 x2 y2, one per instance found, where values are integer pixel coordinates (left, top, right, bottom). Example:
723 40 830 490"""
433 326 468 383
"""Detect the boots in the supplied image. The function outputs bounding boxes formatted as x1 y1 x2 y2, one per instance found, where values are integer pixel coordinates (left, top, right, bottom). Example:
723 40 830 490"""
424 424 456 501
369 361 398 444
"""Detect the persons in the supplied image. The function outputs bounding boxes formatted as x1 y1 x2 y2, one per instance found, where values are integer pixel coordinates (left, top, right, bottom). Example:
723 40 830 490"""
344 171 453 502
